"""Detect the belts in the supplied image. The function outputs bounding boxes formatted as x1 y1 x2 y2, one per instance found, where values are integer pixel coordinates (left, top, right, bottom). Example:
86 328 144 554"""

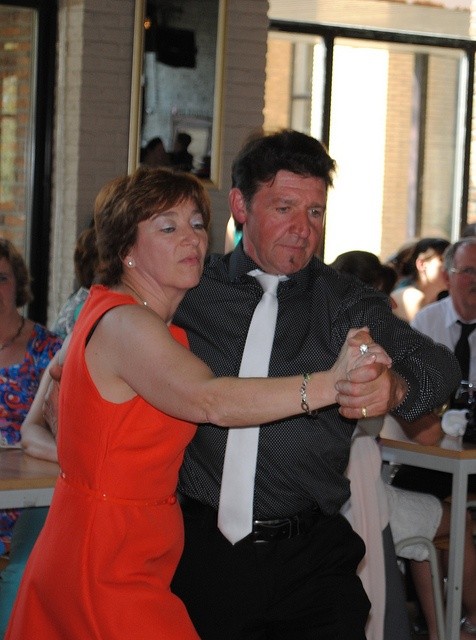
182 494 320 545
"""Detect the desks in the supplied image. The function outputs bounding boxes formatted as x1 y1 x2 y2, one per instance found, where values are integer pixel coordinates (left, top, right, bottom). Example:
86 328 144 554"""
378 438 476 640
0 448 60 510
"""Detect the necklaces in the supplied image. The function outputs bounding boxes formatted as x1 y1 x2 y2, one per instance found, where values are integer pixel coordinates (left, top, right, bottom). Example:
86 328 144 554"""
120 281 151 309
0 315 25 353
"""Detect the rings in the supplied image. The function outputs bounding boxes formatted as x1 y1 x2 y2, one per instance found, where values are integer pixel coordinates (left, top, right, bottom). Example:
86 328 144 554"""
360 406 368 419
359 344 368 355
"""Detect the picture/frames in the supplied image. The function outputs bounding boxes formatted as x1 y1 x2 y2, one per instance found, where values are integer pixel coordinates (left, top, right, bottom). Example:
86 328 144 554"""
127 0 229 193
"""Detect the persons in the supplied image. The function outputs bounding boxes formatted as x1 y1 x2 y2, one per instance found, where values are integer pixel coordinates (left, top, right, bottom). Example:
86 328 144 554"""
139 132 210 179
0 228 99 580
321 236 476 640
3 167 392 640
42 128 461 640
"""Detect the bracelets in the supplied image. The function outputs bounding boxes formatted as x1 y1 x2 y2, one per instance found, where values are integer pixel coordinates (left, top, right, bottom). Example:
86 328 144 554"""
300 372 318 417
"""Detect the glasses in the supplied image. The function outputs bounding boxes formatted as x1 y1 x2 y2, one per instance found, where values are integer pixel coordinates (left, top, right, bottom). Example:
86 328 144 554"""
448 267 475 279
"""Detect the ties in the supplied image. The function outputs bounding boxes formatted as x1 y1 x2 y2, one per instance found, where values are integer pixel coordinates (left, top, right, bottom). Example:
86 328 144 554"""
453 320 476 407
217 268 289 545
